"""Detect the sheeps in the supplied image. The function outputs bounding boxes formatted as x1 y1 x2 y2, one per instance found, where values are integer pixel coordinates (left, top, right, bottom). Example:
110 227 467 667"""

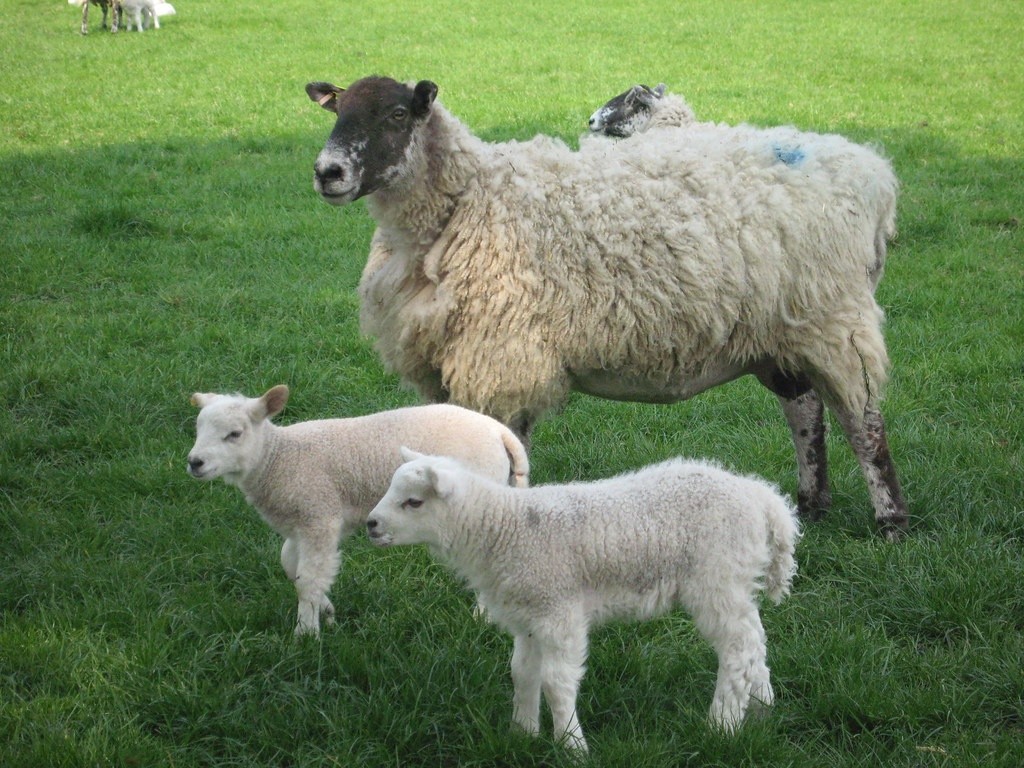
185 384 530 639
365 444 802 768
68 0 176 36
587 83 696 138
305 75 911 544
